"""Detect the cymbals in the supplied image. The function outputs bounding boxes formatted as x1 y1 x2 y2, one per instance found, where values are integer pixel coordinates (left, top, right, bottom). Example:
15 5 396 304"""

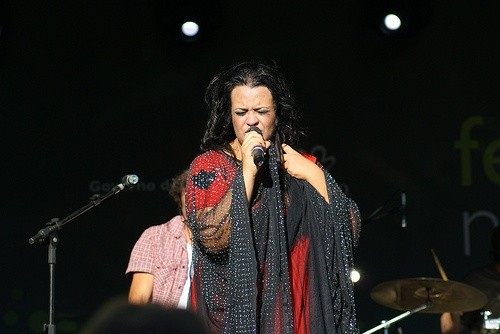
369 276 489 314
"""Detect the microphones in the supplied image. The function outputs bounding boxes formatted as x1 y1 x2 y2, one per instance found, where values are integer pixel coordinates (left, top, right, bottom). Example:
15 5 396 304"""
121 175 139 190
246 126 264 167
400 193 407 228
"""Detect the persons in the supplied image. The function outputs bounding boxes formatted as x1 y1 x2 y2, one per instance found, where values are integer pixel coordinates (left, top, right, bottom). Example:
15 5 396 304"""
185 61 361 334
441 225 500 334
88 304 208 334
126 169 194 310
